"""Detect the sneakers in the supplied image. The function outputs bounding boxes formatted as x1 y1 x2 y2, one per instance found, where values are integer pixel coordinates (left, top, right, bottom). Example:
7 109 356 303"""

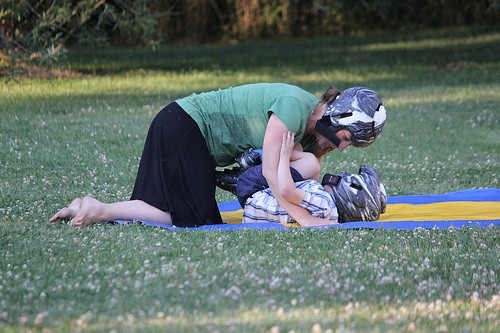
235 148 262 171
215 167 244 195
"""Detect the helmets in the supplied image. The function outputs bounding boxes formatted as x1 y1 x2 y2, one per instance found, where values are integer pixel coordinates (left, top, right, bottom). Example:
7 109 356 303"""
315 86 387 147
321 162 387 223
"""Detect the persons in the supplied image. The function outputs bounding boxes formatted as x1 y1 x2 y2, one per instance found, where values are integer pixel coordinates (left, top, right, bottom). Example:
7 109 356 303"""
49 83 387 228
215 131 387 223
